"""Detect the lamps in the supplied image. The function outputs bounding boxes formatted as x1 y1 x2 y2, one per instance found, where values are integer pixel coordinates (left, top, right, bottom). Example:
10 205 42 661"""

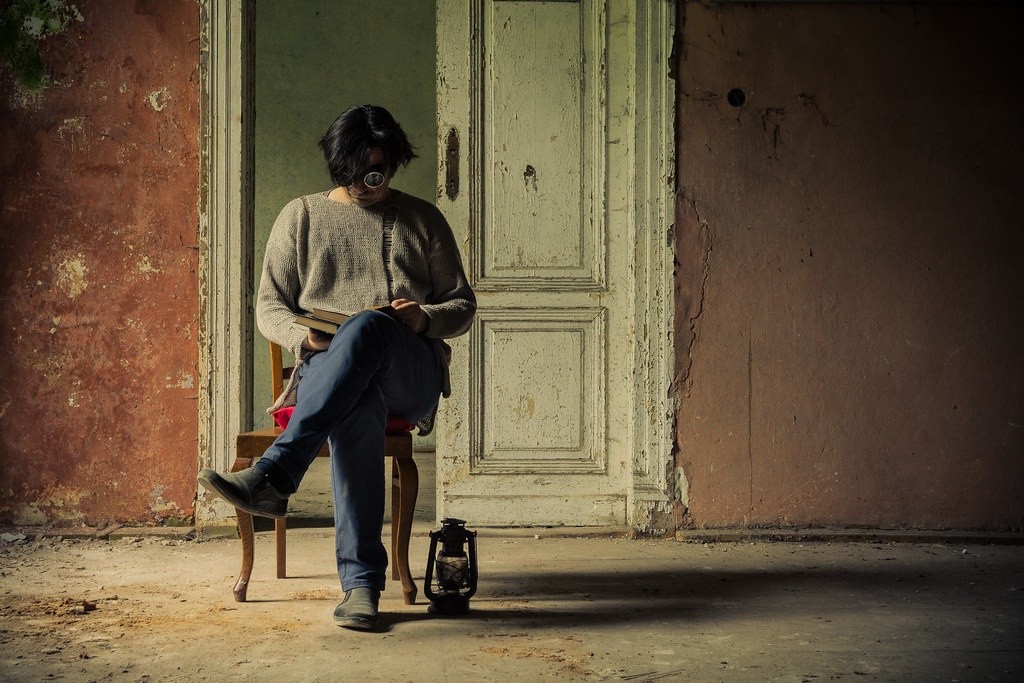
423 516 478 616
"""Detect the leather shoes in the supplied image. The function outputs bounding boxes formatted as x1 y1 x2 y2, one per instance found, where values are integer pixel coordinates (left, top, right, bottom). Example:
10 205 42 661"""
197 465 288 517
333 585 380 629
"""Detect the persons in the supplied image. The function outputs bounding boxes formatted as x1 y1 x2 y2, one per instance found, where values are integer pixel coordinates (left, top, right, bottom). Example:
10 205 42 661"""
197 104 476 630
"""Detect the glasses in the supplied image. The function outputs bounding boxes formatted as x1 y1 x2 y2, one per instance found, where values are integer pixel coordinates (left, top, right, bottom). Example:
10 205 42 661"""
335 169 387 189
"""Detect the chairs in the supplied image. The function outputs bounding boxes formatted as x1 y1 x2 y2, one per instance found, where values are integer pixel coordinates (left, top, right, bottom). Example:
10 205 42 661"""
233 343 421 606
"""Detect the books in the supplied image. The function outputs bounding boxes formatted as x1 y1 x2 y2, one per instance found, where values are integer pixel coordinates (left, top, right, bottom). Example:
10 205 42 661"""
293 313 339 334
311 305 392 326
